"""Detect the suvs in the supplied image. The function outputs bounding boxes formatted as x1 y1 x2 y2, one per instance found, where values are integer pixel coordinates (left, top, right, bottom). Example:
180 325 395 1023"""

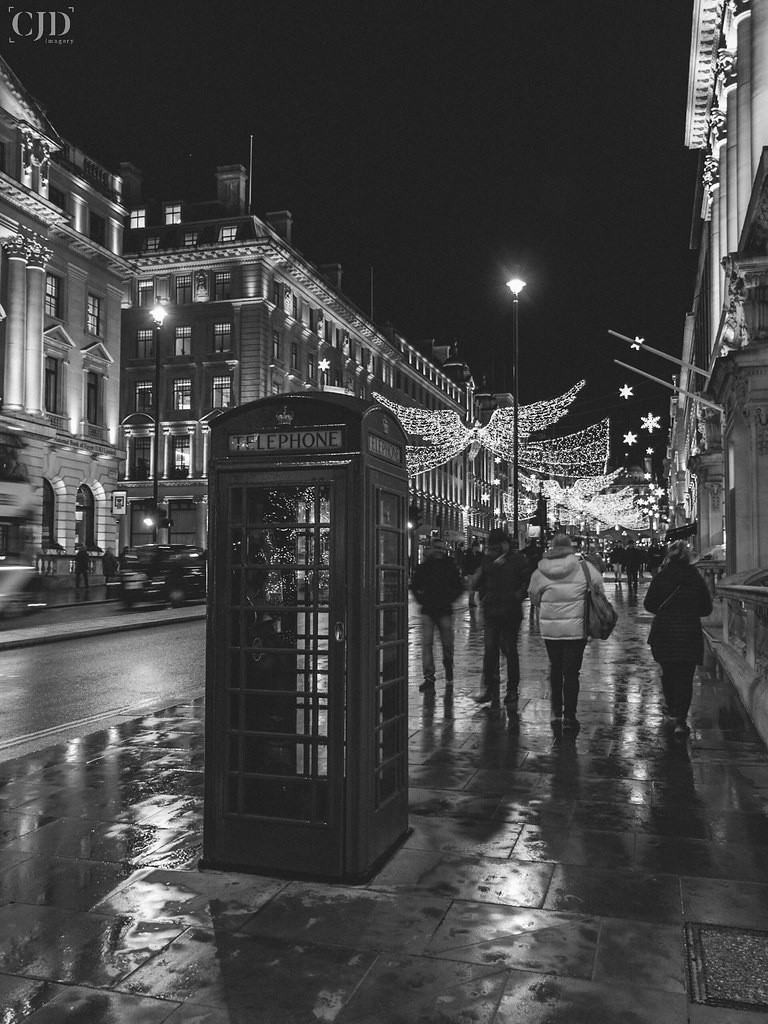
114 542 206 610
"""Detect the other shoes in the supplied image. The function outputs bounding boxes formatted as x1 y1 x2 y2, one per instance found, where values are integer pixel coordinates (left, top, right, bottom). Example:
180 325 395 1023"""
446 666 453 682
504 691 519 703
475 690 501 706
419 680 435 692
563 713 580 727
550 708 562 727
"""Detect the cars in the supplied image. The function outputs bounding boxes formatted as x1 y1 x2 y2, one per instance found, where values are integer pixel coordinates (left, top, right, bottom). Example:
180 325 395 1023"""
0 553 47 619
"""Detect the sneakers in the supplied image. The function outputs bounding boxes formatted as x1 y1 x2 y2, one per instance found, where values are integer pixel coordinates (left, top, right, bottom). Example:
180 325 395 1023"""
661 706 690 734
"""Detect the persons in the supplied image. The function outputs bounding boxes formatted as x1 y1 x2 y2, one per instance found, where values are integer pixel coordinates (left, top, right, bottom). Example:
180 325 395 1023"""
643 539 713 735
621 540 641 590
410 538 464 691
102 551 116 585
465 541 480 608
75 545 90 590
470 528 529 705
527 533 606 726
612 540 626 581
519 538 542 573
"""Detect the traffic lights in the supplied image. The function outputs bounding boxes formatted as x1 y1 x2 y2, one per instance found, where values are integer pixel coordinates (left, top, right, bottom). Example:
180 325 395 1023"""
408 506 417 531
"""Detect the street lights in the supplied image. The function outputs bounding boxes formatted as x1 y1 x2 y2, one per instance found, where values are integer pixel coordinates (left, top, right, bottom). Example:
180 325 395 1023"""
151 305 166 544
507 277 527 553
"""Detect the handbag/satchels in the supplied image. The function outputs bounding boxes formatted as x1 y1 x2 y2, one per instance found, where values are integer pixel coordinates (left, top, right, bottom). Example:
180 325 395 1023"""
579 560 618 640
